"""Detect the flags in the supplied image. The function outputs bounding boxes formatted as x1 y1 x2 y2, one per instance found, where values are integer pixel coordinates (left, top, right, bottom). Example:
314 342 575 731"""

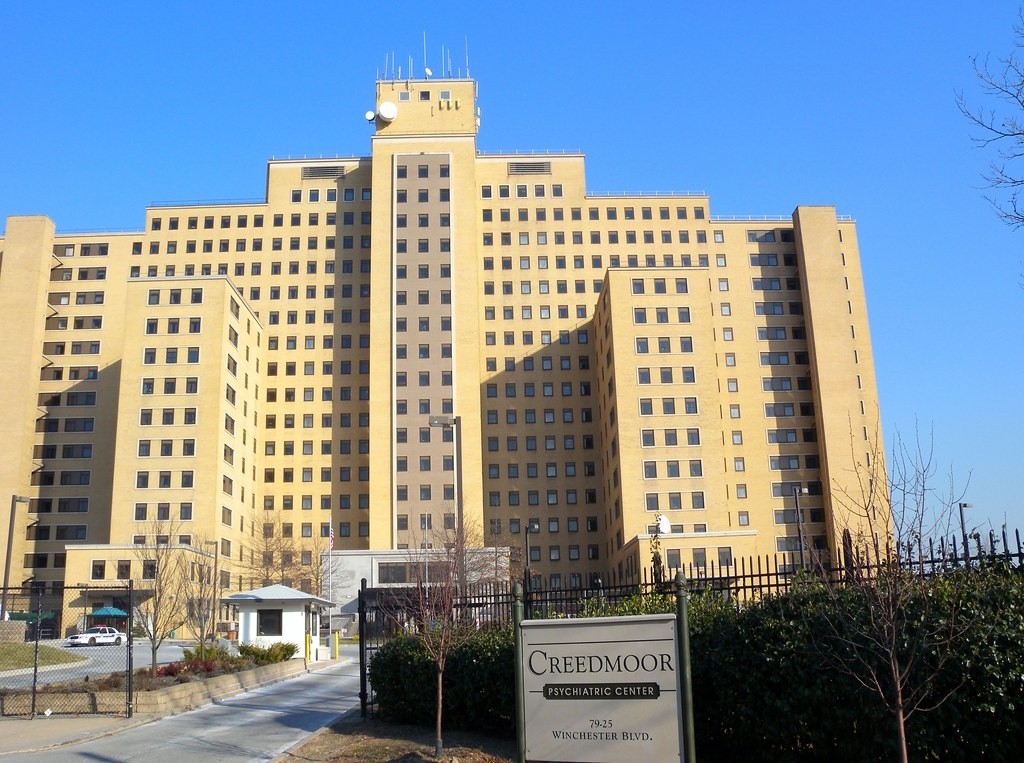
331 527 333 549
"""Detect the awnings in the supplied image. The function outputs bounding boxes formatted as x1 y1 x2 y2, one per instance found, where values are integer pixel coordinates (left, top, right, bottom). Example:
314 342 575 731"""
9 613 55 625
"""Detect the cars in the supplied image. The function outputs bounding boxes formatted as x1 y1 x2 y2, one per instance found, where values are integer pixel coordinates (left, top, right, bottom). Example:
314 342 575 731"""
67 624 128 647
320 622 330 629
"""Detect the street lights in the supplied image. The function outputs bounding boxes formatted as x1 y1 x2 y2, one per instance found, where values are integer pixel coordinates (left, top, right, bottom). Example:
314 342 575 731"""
205 540 218 643
0 495 31 619
428 415 468 633
794 487 810 590
77 582 89 632
525 523 540 620
959 503 974 568
